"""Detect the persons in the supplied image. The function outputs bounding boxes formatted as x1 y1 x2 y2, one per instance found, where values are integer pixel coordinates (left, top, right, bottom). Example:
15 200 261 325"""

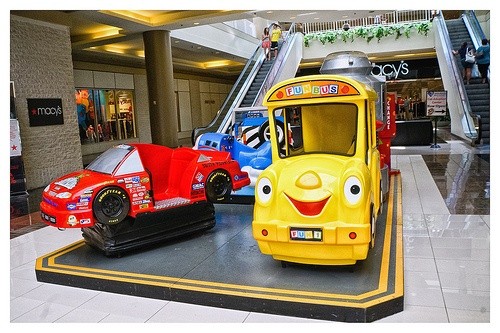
122 118 131 140
403 96 411 121
374 15 382 24
452 42 475 85
270 23 281 60
262 27 272 61
474 38 490 84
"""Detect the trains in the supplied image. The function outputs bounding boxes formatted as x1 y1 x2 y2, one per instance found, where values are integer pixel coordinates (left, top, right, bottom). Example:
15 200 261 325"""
252 51 384 267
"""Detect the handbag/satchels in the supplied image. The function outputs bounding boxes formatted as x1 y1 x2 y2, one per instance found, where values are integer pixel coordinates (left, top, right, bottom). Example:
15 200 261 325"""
471 64 482 78
465 47 475 63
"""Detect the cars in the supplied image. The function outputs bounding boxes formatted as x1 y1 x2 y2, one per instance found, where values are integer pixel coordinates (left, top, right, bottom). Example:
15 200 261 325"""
37 142 252 229
197 114 286 199
